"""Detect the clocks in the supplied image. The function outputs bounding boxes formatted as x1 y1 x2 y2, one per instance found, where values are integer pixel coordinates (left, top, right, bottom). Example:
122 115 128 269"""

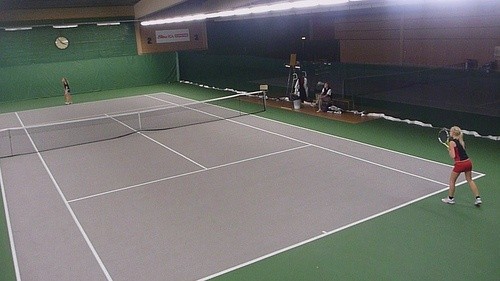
54 36 69 50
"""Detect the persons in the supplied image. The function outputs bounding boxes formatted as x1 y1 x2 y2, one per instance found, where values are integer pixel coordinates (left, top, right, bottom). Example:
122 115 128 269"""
441 126 483 206
311 82 333 114
61 77 72 105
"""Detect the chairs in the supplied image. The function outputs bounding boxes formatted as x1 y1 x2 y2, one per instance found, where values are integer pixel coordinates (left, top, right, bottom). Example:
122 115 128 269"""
258 85 269 99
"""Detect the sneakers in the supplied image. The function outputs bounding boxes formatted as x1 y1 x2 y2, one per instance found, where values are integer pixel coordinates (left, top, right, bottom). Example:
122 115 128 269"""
474 198 482 205
442 196 455 204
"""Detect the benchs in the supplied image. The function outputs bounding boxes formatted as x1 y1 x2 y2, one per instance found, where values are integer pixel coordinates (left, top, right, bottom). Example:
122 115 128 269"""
318 98 354 112
